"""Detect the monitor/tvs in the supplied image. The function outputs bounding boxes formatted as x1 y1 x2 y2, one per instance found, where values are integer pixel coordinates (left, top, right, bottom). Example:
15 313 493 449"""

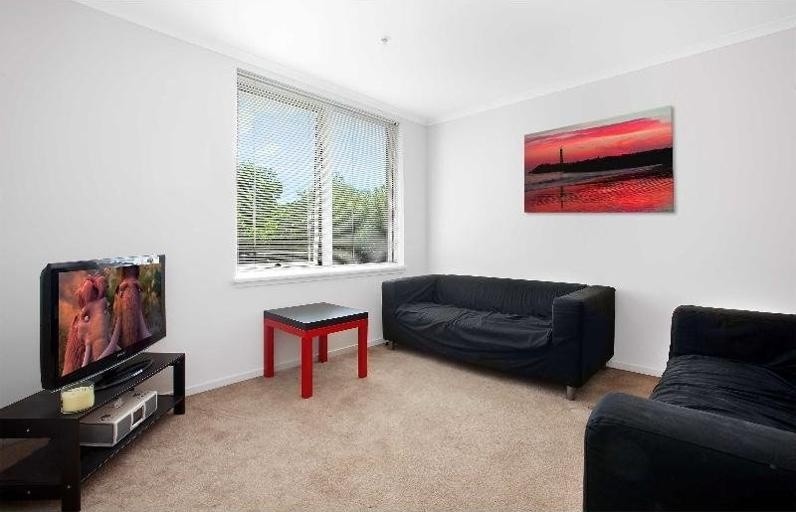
40 254 166 394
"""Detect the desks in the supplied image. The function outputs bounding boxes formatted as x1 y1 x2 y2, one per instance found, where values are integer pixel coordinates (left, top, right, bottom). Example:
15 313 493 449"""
0 352 185 511
264 302 368 398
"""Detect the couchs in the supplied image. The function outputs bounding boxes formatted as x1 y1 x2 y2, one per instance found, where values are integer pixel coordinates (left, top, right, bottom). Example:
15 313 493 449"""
583 305 796 512
382 274 615 400
